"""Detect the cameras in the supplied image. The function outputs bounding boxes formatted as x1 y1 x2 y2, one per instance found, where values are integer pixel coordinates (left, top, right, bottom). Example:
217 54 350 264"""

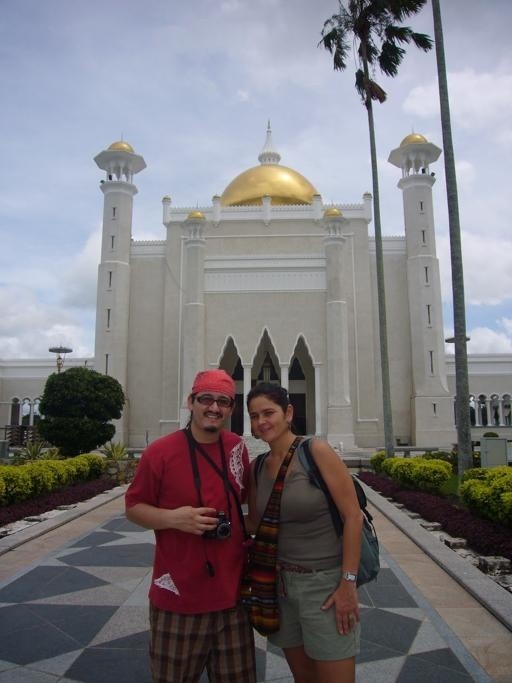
201 511 231 539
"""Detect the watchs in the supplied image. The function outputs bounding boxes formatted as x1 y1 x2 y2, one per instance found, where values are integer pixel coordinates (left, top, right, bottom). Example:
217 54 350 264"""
339 570 359 585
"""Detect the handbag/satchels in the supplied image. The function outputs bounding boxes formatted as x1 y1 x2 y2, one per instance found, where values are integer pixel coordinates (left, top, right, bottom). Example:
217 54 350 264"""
350 474 380 586
240 538 281 635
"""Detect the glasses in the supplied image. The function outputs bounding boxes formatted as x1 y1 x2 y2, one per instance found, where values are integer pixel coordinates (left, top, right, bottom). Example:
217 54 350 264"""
193 393 234 410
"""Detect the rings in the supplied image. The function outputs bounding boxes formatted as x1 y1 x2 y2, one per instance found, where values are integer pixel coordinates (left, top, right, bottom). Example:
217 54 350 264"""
349 615 357 625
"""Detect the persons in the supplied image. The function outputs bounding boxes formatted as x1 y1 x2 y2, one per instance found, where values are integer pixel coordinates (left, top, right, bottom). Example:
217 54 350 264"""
124 369 256 682
246 381 365 683
